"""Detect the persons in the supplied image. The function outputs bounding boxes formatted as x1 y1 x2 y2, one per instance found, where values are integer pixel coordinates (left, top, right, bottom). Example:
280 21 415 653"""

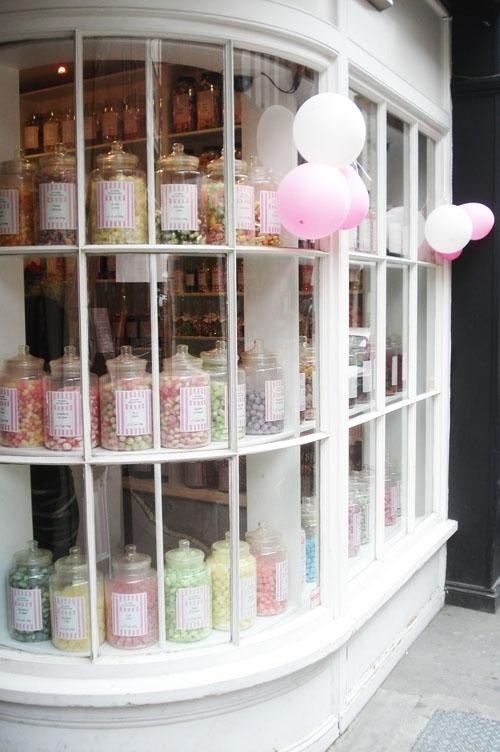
24 269 108 561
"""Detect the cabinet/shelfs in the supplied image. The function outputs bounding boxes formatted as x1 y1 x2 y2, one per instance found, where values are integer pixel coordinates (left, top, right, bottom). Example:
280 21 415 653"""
3 237 430 667
20 121 366 511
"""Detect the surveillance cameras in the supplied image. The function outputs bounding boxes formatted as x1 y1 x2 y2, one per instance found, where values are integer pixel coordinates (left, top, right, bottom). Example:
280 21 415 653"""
235 69 260 93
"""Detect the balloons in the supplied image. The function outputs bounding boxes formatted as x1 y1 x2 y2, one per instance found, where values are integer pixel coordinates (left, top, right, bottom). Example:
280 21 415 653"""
274 161 351 241
423 202 496 260
334 166 370 230
291 91 367 171
255 105 299 183
384 204 429 261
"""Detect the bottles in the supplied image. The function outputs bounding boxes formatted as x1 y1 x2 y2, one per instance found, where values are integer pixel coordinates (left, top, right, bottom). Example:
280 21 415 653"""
44 346 100 451
164 538 212 643
250 158 281 248
242 337 286 434
98 344 154 452
161 344 211 449
300 188 401 589
156 142 206 243
245 520 288 616
86 140 149 245
200 339 247 443
1 344 46 448
203 148 255 246
8 538 53 645
207 530 258 632
106 544 158 652
0 142 36 246
50 546 107 651
33 143 78 245
